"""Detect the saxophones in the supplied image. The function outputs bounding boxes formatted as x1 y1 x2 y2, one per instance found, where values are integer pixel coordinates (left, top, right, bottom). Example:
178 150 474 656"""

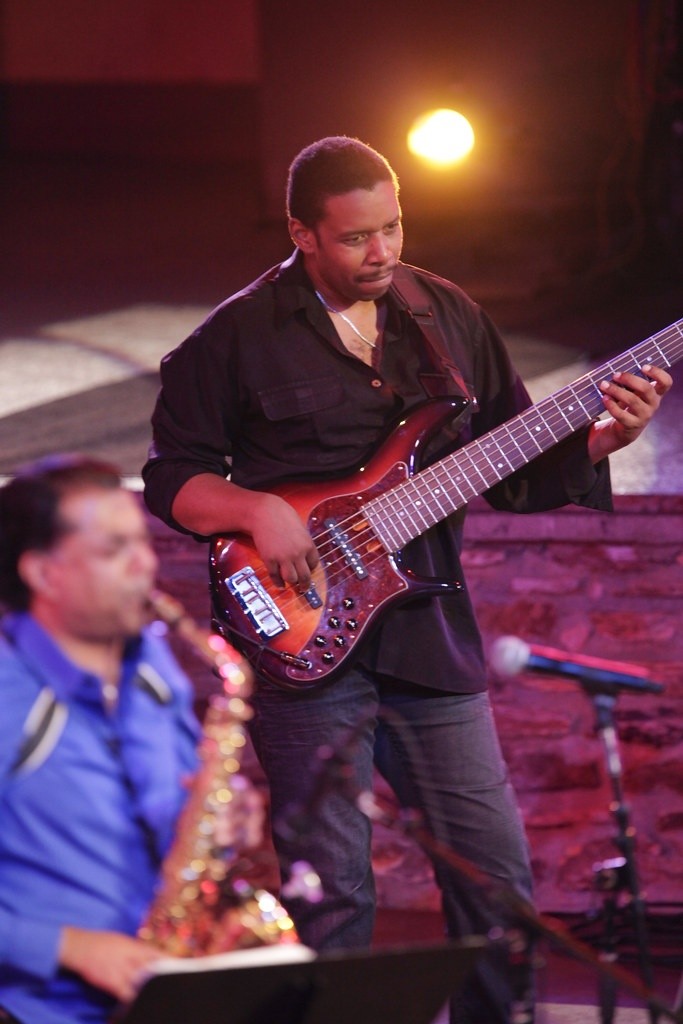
113 584 299 1022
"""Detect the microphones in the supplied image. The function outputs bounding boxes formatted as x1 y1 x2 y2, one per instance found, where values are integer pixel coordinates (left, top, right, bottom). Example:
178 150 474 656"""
488 636 666 701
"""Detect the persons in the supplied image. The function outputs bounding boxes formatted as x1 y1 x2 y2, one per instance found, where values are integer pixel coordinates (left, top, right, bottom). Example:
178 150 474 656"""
142 138 672 1024
0 456 261 1024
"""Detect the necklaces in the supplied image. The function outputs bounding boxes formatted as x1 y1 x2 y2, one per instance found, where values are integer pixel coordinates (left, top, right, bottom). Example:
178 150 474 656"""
313 289 382 352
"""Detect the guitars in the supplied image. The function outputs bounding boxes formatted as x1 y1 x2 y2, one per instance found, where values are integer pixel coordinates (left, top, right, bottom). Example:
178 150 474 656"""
210 320 683 690
